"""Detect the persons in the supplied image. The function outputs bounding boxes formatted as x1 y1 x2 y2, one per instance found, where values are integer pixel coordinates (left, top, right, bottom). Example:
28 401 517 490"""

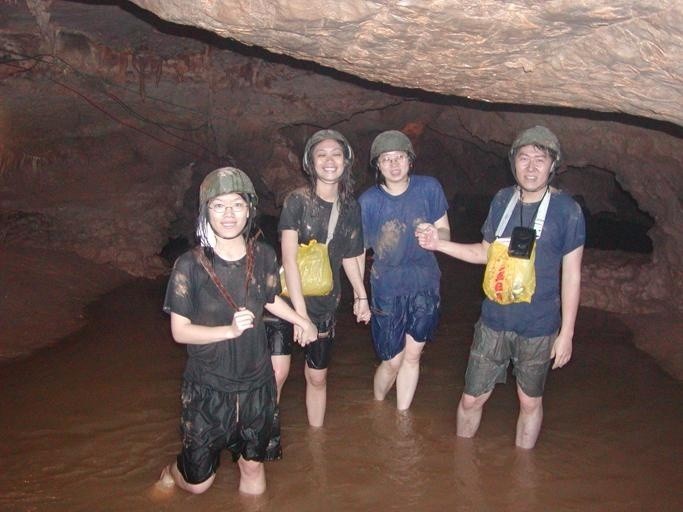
418 125 585 449
353 131 451 410
263 130 371 426
159 167 317 494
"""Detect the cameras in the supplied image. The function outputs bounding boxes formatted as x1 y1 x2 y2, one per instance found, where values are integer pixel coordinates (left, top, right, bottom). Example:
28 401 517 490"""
509 227 536 258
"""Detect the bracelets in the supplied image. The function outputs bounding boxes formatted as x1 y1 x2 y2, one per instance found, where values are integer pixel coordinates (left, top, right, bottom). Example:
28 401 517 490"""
354 297 359 300
359 298 368 300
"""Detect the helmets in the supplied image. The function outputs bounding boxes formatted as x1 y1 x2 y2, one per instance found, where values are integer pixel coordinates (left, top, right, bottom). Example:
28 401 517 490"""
370 131 415 168
510 126 561 163
200 168 258 205
303 129 353 172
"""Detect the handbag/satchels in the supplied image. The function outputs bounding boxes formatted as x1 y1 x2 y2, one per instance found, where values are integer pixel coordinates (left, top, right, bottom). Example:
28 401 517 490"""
482 185 550 304
279 195 341 298
